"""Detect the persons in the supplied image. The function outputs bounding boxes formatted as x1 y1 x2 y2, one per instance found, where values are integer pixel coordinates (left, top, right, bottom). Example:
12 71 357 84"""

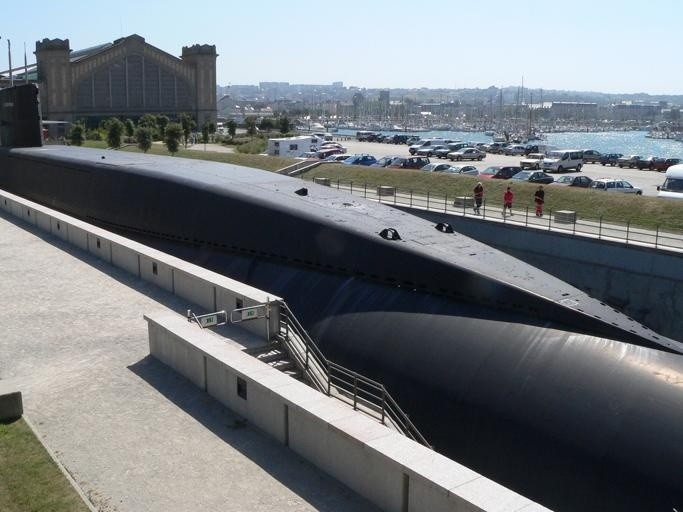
502 187 514 215
535 186 545 217
473 182 484 215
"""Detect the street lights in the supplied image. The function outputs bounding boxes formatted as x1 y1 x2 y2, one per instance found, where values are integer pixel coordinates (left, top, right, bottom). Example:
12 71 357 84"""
0 35 13 89
21 40 28 85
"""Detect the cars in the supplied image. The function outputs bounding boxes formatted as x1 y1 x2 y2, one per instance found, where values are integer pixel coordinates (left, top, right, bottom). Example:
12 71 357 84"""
217 122 224 132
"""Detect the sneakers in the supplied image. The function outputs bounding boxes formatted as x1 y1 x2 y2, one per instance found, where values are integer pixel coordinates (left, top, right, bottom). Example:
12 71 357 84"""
540 213 543 217
536 213 539 217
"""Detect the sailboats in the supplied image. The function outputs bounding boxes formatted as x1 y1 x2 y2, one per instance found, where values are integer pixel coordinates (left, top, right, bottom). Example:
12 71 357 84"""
323 95 486 133
485 76 547 144
